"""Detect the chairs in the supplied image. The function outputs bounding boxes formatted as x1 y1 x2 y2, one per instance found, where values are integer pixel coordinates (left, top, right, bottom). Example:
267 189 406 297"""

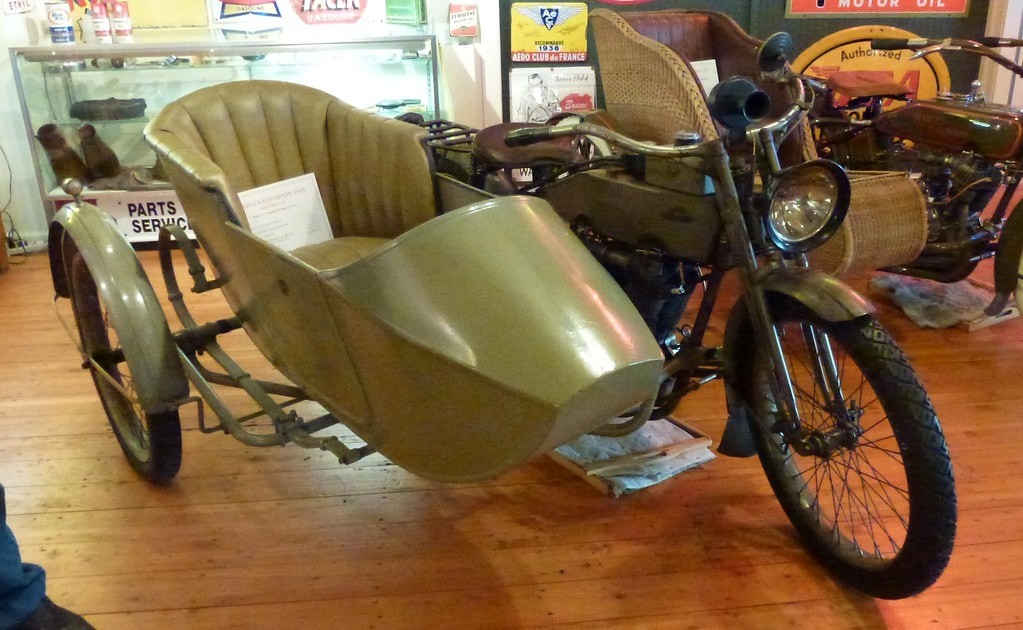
587 8 819 165
141 79 441 269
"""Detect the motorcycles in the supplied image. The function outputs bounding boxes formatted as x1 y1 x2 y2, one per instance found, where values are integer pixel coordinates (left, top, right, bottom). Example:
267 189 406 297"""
796 36 1023 322
48 5 957 601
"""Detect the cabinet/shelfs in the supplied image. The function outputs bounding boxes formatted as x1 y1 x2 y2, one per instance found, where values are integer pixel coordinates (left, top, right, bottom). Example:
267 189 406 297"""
6 33 440 242
